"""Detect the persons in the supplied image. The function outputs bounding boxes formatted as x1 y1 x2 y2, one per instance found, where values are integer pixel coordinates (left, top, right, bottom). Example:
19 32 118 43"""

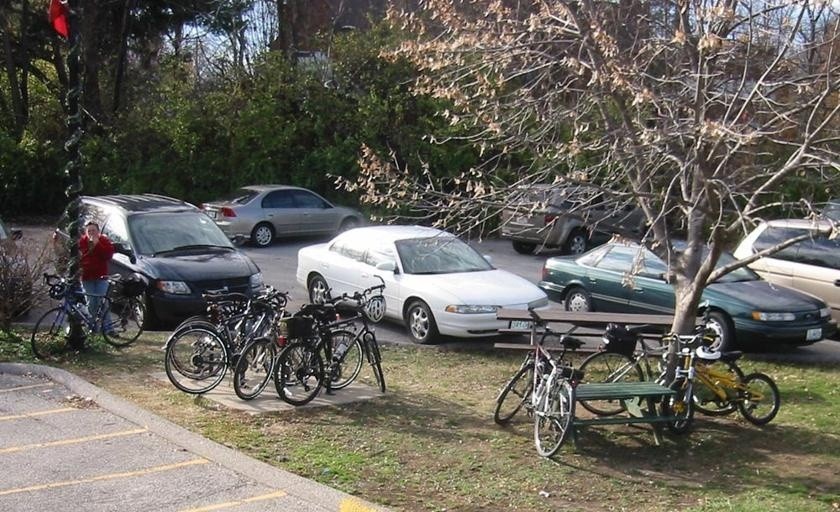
79 222 120 339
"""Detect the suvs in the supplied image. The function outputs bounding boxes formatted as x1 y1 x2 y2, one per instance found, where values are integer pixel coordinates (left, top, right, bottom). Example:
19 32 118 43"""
499 182 636 258
52 193 266 330
537 236 832 361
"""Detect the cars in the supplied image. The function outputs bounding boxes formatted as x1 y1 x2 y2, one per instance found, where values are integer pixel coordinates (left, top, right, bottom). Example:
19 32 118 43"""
1 220 34 321
295 225 548 346
732 219 840 343
199 184 366 249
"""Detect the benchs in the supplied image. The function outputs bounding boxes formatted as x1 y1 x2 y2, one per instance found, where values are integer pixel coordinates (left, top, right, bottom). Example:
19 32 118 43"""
554 380 685 451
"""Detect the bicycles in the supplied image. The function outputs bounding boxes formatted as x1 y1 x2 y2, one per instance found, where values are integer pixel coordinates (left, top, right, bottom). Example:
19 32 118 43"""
573 298 745 416
493 305 587 457
660 333 780 436
30 268 149 361
162 274 387 407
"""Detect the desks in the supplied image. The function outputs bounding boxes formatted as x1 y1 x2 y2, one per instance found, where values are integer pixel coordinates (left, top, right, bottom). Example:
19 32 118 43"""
494 308 705 363
